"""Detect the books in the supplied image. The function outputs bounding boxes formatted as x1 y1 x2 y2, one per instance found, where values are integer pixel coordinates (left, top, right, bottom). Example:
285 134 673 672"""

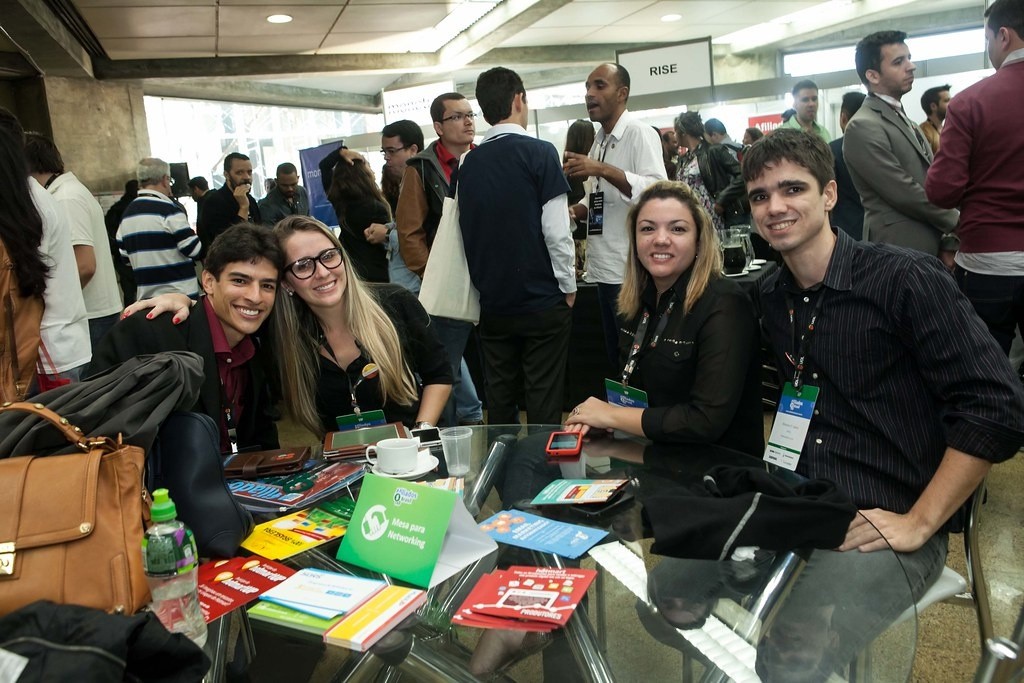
246 567 427 652
224 420 410 512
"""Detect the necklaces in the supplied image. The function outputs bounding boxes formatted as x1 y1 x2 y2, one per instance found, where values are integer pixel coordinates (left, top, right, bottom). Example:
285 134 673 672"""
218 367 245 454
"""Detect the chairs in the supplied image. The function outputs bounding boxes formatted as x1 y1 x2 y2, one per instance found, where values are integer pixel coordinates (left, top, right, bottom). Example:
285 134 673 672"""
846 471 996 683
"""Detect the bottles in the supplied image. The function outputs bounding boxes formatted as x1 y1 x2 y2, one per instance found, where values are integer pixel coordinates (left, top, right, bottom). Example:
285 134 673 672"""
140 488 208 649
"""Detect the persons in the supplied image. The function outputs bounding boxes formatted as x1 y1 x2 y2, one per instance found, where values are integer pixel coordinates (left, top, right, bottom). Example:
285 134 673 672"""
0 108 123 406
394 66 578 509
269 217 454 439
842 29 962 270
256 162 312 222
561 179 766 563
201 151 262 254
365 119 485 426
649 84 955 267
924 0 1024 382
80 222 284 453
185 176 216 238
102 157 202 302
563 63 668 408
319 145 390 283
775 78 831 151
646 129 1024 683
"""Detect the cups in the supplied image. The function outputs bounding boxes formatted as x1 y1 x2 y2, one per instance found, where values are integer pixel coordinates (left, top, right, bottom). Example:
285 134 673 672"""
728 225 756 268
439 425 473 477
366 438 418 474
719 228 748 275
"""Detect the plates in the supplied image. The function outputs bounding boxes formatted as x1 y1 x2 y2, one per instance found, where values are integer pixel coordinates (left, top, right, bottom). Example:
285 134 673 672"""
371 455 438 481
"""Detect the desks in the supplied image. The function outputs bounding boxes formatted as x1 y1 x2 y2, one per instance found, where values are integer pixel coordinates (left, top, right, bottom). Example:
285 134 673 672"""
202 423 920 683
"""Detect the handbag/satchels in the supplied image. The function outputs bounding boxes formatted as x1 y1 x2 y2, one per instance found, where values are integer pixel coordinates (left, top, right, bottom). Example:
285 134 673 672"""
0 399 155 615
419 182 481 325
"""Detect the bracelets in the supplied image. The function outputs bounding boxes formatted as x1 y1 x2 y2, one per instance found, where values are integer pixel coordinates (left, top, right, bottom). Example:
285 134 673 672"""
385 228 391 242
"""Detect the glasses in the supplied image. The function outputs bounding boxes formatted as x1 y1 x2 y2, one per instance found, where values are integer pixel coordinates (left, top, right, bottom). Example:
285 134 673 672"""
440 113 478 122
167 176 175 186
283 248 344 280
381 147 408 154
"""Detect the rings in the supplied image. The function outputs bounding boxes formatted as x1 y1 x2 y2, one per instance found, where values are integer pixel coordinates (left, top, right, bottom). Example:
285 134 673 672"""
572 406 579 414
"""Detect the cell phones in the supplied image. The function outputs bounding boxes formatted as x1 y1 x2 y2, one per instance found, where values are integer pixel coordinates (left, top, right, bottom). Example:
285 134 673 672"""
409 427 442 448
545 431 583 456
